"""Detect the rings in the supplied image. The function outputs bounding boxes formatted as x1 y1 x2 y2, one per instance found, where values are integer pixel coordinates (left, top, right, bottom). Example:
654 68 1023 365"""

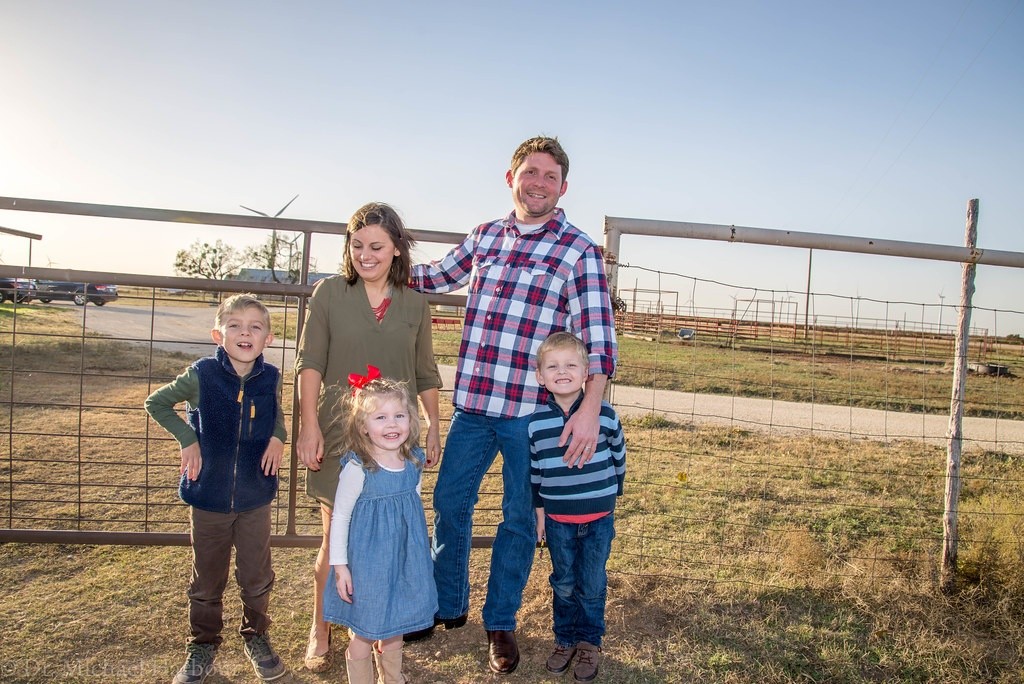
586 447 591 450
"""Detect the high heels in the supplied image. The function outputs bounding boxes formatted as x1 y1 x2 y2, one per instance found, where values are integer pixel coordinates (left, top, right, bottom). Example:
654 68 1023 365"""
306 623 335 671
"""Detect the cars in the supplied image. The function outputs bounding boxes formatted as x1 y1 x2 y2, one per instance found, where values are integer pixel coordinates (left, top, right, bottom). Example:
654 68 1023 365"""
0 276 38 305
37 278 119 306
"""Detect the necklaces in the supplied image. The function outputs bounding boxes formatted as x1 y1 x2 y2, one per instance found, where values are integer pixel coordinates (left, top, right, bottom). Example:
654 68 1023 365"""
371 288 392 323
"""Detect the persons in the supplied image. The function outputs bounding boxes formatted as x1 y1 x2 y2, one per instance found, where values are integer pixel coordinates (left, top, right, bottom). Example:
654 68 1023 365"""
527 332 627 681
400 137 617 672
145 295 287 684
322 377 439 684
296 203 444 672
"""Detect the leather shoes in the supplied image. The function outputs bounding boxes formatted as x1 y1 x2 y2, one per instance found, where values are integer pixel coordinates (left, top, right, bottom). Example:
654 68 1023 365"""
487 629 520 675
404 616 467 641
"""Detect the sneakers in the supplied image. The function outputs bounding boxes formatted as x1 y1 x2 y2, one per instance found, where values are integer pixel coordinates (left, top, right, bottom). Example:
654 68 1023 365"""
244 631 287 681
546 641 602 682
171 639 217 684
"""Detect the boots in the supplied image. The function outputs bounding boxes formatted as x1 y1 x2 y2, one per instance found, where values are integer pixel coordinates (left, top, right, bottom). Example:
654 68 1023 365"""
344 642 407 683
